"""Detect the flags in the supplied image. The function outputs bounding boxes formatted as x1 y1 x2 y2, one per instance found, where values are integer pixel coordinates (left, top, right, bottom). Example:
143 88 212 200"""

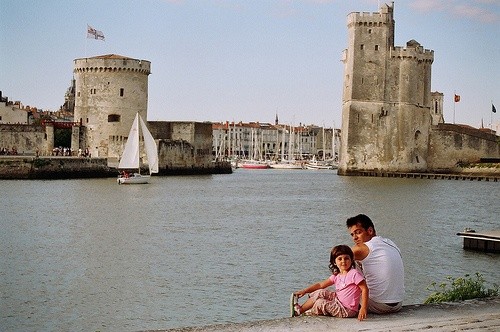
86 25 105 41
455 95 461 101
492 105 497 113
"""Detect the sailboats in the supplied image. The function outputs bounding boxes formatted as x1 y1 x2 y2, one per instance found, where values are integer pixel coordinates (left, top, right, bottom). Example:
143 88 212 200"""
219 123 340 169
115 111 159 184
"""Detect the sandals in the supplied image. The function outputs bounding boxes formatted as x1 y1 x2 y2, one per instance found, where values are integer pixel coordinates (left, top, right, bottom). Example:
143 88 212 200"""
291 293 300 317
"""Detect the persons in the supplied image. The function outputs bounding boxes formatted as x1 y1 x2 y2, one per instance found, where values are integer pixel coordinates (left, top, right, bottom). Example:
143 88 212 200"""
36 147 39 157
0 145 18 156
53 146 89 158
118 169 135 178
334 214 405 314
290 244 369 322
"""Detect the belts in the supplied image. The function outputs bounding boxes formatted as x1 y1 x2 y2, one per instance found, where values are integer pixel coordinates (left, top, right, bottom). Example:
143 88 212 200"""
385 303 398 306
